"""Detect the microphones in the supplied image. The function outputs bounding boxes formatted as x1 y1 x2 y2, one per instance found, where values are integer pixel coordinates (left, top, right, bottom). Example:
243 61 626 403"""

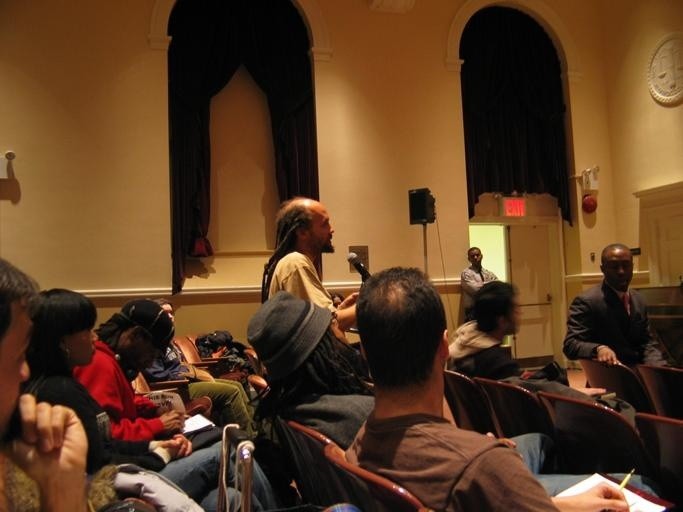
347 253 372 282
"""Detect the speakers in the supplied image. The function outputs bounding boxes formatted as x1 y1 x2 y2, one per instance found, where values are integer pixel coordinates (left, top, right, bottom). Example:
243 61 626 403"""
409 188 435 224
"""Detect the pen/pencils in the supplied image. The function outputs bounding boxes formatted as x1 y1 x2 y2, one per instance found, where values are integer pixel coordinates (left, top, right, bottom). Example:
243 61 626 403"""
605 469 636 511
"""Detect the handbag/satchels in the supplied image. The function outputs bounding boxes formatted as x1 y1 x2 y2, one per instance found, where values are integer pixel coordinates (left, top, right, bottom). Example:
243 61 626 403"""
593 395 635 429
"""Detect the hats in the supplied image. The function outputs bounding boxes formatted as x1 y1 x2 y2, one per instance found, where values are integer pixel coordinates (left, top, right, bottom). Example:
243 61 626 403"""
247 291 333 383
120 299 175 351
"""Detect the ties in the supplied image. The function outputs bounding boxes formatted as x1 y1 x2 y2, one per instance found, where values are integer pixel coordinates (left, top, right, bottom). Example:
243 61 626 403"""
478 270 484 281
623 292 629 314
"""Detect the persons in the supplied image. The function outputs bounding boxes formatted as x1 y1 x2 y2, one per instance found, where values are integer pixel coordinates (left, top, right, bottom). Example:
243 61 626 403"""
458 245 503 322
247 267 682 511
0 258 357 512
561 243 670 367
259 197 360 331
